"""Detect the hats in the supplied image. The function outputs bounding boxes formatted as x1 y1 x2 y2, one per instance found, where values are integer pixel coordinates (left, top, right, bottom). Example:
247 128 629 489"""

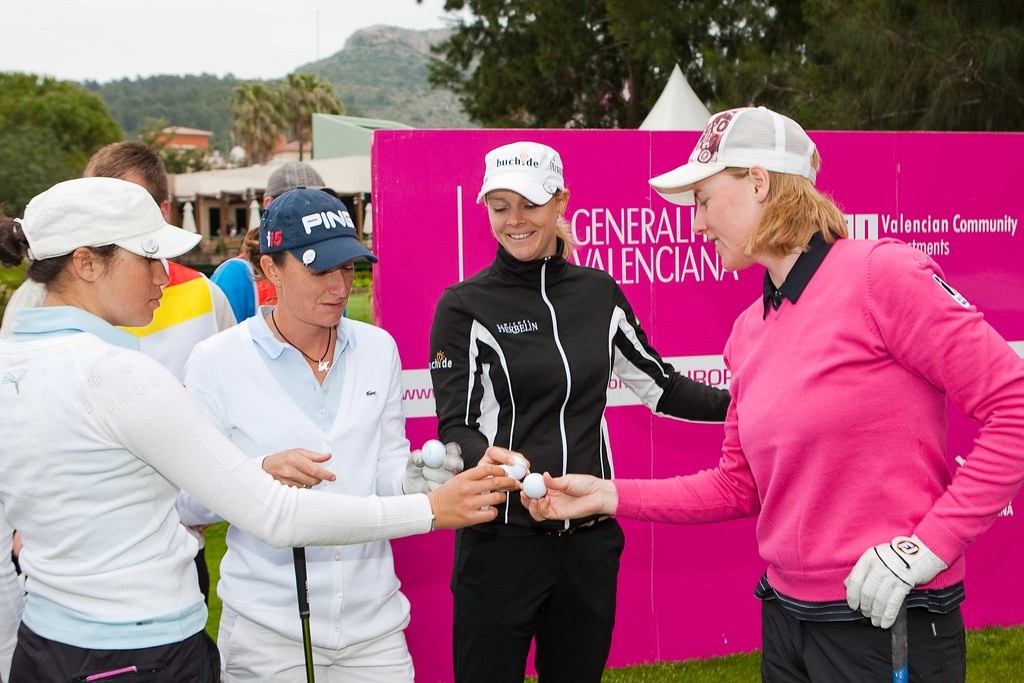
476 141 564 205
259 186 378 273
264 162 338 198
14 177 203 258
648 106 817 205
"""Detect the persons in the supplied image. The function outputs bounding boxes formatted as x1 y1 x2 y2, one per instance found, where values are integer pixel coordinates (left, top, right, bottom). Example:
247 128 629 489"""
0 175 518 683
213 161 347 324
520 105 1024 683
217 223 236 237
177 187 465 683
0 138 239 610
429 141 731 683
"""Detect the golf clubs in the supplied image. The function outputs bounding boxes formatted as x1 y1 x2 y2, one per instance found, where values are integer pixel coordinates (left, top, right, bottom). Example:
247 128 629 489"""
891 597 908 683
293 546 315 683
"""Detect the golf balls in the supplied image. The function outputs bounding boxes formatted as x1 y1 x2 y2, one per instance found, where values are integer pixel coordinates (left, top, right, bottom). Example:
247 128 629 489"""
421 439 446 469
522 472 547 499
493 465 516 492
503 455 526 480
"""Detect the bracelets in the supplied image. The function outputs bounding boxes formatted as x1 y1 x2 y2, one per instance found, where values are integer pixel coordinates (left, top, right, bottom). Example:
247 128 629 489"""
431 514 435 532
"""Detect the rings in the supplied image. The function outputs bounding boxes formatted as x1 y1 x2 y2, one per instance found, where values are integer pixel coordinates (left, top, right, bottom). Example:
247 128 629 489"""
303 484 306 488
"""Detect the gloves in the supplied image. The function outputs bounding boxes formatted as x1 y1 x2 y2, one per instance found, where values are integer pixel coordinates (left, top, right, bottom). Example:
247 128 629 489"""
844 533 948 629
401 442 465 494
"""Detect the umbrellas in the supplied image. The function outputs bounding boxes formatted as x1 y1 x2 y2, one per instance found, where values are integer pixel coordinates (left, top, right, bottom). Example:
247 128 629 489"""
363 203 372 240
181 199 198 233
249 200 261 231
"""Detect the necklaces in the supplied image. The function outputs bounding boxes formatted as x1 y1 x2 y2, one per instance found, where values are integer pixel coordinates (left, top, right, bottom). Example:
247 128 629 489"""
271 309 332 372
